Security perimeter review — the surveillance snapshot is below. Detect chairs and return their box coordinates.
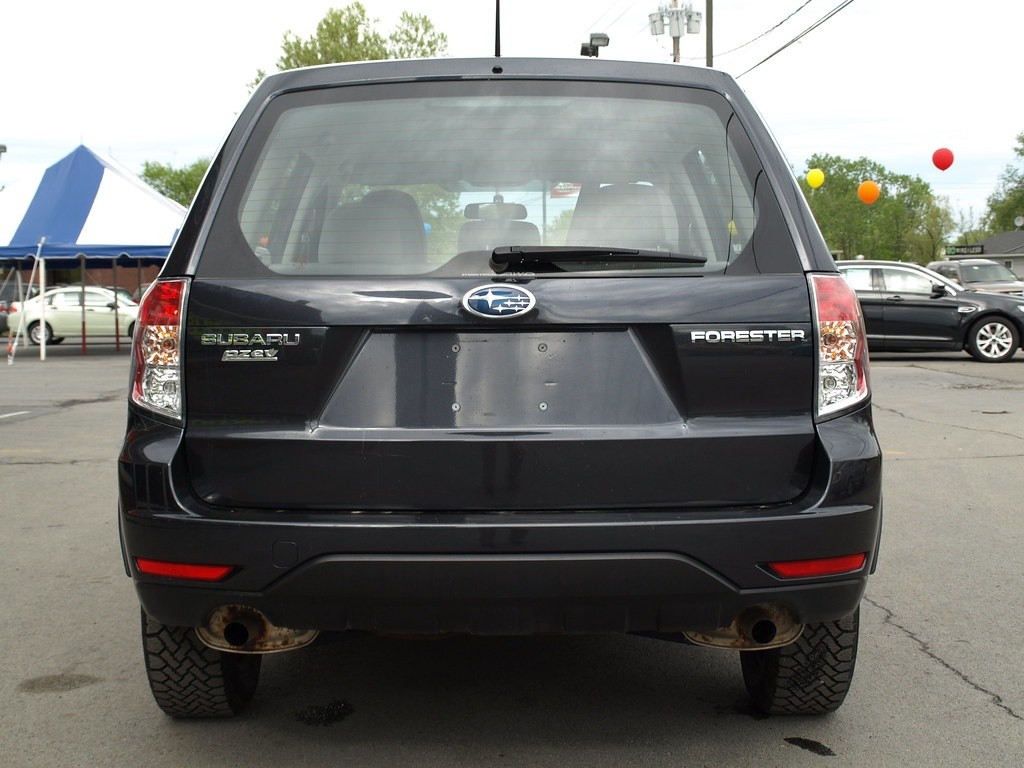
[317,190,427,270]
[570,182,682,254]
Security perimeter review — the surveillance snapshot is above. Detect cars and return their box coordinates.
[105,285,135,303]
[133,281,154,303]
[6,286,140,345]
[834,259,1024,364]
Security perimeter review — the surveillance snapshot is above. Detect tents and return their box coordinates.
[0,145,189,363]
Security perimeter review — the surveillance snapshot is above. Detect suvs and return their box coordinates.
[118,55,884,722]
[926,257,1024,300]
[0,281,40,337]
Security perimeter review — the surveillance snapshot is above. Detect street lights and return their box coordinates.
[648,0,702,65]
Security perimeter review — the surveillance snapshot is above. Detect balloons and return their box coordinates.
[858,181,881,205]
[806,169,825,189]
[933,148,954,171]
